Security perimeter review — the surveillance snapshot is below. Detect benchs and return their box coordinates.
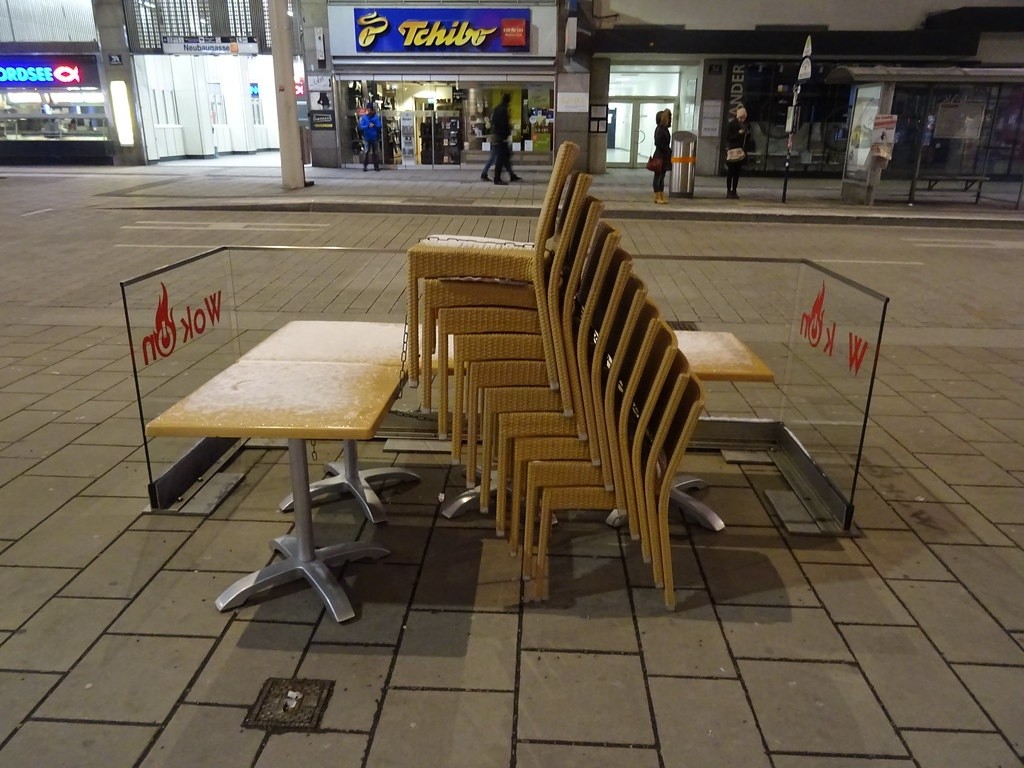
[923,174,990,192]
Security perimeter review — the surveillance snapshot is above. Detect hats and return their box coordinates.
[736,104,747,118]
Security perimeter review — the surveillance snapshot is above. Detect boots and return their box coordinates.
[655,191,668,204]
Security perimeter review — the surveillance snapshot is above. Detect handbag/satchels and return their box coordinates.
[726,148,745,161]
[646,156,663,172]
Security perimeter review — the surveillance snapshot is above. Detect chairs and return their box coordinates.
[405,141,711,616]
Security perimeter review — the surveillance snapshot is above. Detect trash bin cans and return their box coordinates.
[669,131,697,198]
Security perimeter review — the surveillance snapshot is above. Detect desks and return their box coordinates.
[603,327,776,531]
[144,319,431,624]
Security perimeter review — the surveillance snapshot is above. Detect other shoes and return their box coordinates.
[480,172,491,181]
[510,175,523,182]
[374,166,380,171]
[363,166,367,171]
[493,175,508,185]
[726,189,739,199]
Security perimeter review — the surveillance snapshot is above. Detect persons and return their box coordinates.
[652,108,672,205]
[359,102,382,172]
[724,104,752,200]
[480,92,524,185]
[69,119,76,132]
[42,118,58,138]
